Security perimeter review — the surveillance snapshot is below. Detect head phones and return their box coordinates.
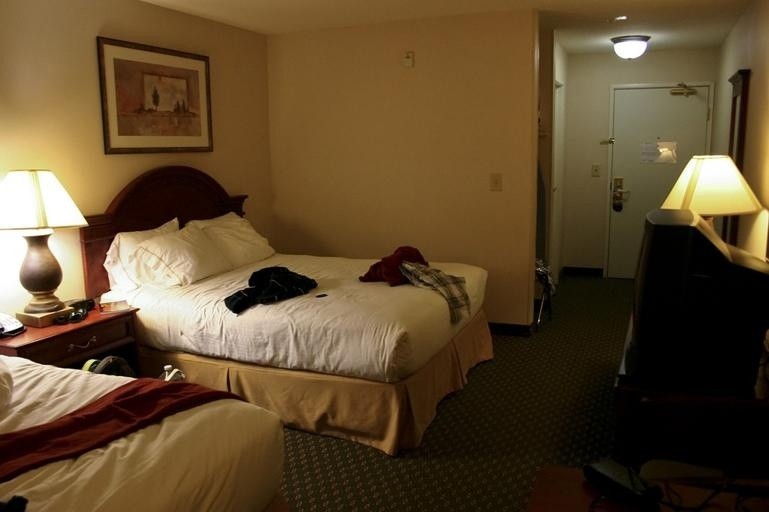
[52,307,88,325]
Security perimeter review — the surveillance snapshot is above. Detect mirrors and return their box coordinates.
[721,67,750,245]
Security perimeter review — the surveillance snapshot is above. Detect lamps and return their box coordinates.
[611,36,651,61]
[0,171,90,329]
[659,155,764,231]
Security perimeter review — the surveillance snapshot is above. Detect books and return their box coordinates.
[97,299,130,315]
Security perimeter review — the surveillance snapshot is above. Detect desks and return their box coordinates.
[526,463,768,512]
[612,312,768,473]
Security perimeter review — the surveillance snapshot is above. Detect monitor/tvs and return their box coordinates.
[625,209,769,396]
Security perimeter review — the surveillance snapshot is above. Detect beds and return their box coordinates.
[79,166,495,458]
[0,354,290,512]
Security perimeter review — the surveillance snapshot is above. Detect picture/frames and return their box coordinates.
[96,37,215,155]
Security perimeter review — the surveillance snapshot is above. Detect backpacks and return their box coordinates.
[81,357,139,379]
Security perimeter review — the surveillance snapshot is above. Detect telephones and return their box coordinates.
[0,312,24,338]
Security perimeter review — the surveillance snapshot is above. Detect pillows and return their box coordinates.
[103,210,277,291]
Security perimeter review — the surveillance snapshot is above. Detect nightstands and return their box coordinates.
[0,305,139,368]
